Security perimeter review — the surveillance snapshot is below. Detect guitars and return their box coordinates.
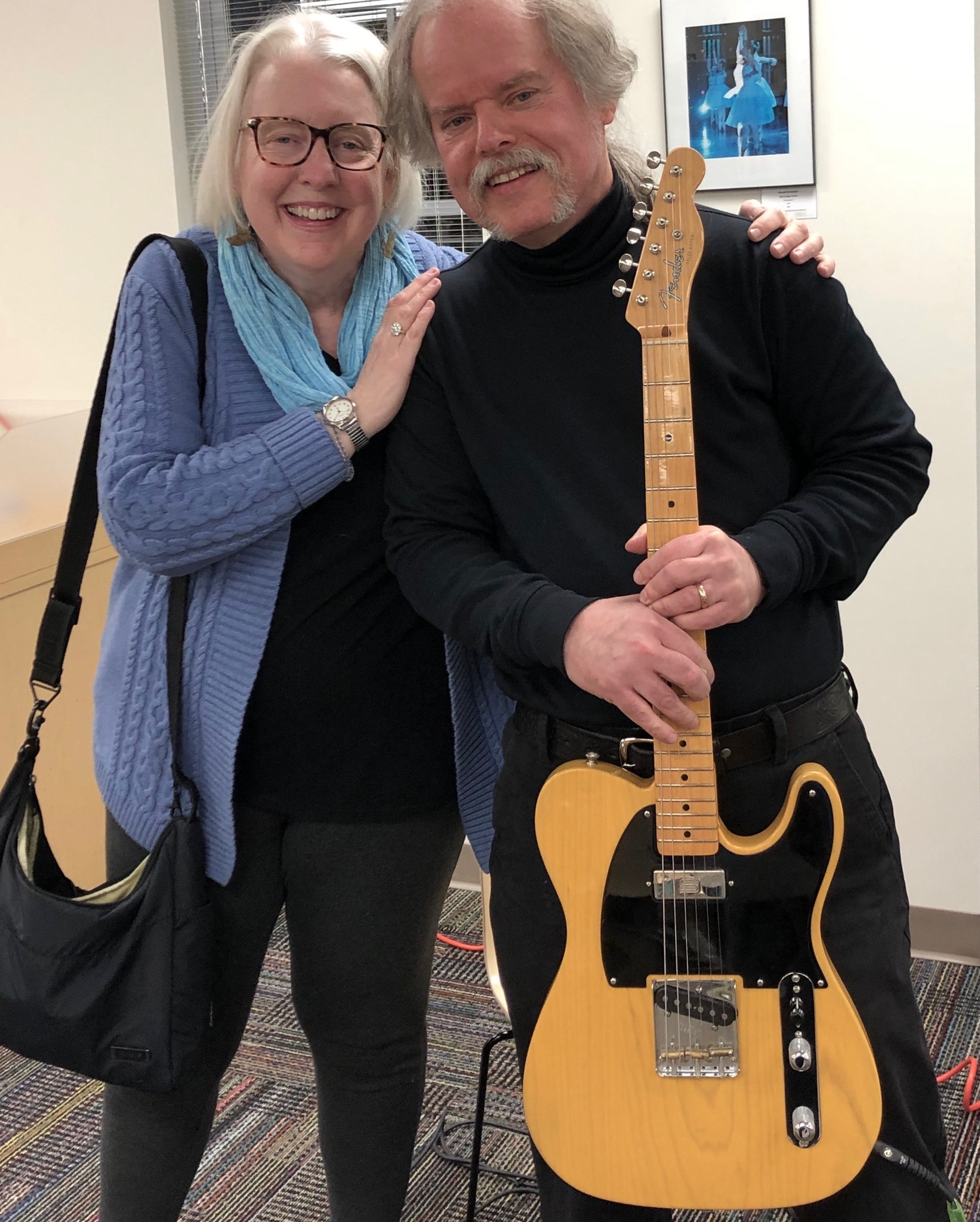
[517,144,881,1211]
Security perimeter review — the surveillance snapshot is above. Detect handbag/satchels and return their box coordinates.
[1,700,211,1092]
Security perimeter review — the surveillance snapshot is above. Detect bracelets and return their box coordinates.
[332,427,354,482]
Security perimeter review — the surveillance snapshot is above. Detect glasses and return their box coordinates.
[238,115,390,171]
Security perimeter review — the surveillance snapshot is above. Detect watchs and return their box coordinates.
[321,395,367,451]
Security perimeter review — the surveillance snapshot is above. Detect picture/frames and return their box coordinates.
[660,0,815,192]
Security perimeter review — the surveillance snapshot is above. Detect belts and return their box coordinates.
[516,671,857,779]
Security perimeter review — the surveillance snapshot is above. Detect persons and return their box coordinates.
[93,11,836,1222]
[368,0,947,1222]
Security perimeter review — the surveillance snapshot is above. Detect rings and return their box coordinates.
[390,322,406,335]
[696,583,708,608]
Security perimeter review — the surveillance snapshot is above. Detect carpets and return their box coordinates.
[1,882,980,1221]
